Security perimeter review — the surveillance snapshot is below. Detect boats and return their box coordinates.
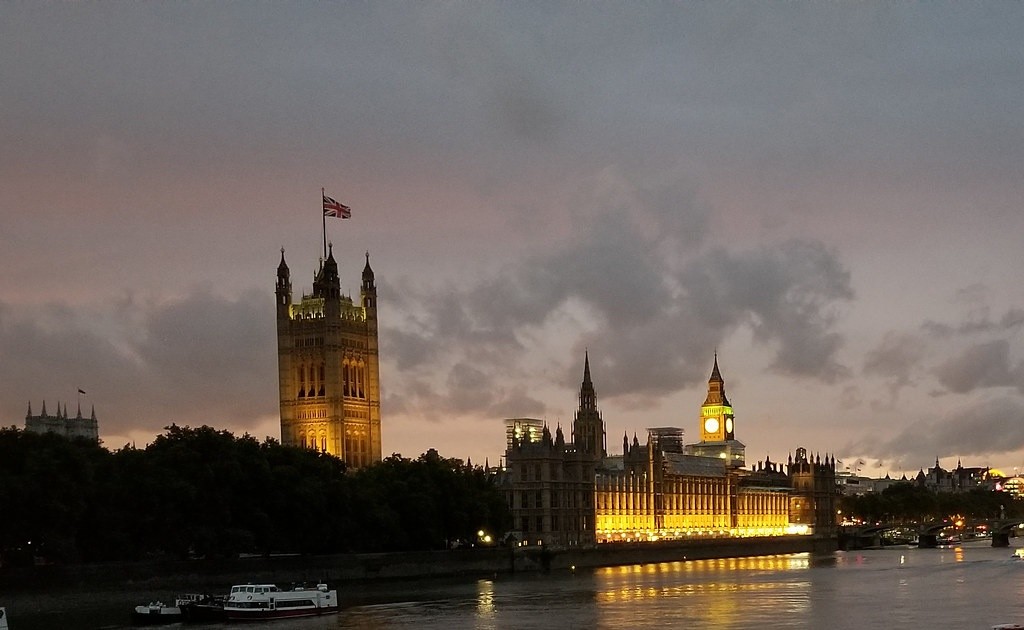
[178,596,227,625]
[134,594,203,624]
[223,583,338,622]
[908,540,919,545]
[939,535,961,545]
[1010,549,1024,560]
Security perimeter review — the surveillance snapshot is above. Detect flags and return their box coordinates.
[324,196,351,219]
[78,389,85,394]
